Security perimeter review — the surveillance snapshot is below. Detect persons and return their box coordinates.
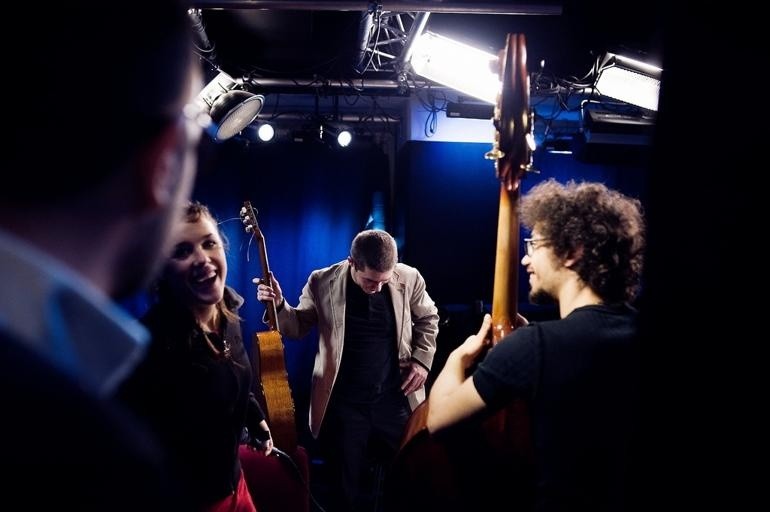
[426,176,683,510]
[0,0,209,510]
[141,195,274,512]
[252,228,440,511]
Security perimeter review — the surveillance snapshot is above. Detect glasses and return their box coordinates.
[523,237,551,256]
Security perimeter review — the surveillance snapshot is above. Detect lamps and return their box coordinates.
[592,52,664,112]
[187,50,356,154]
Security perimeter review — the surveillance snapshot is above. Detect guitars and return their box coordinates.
[484,32,531,348]
[240,200,295,440]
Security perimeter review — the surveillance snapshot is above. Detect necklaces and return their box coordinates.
[202,329,235,366]
